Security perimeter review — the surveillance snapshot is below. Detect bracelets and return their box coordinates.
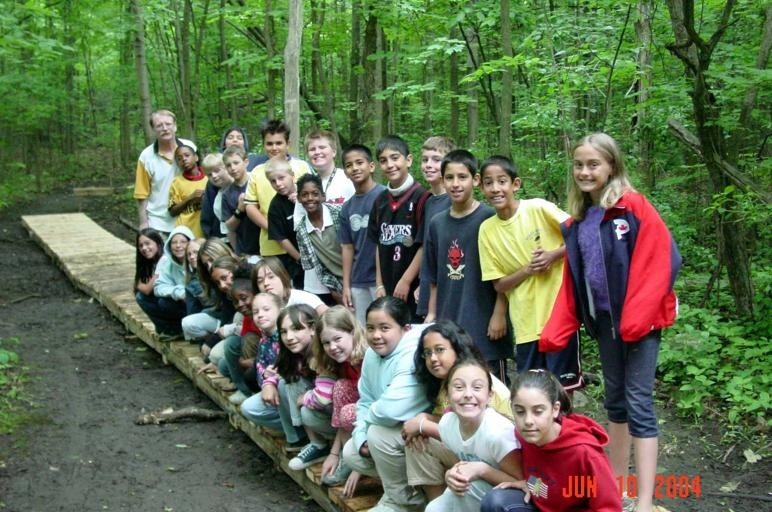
[419,418,429,435]
[375,284,384,291]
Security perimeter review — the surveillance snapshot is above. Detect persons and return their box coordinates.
[339,144,389,327]
[420,149,514,388]
[480,368,623,512]
[477,154,585,413]
[402,318,516,502]
[367,134,428,323]
[132,109,355,472]
[413,135,454,316]
[424,357,524,512]
[559,131,684,512]
[308,304,372,498]
[342,294,434,512]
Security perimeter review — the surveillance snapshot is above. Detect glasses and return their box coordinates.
[420,346,449,359]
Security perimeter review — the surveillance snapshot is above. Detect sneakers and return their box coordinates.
[353,478,384,497]
[158,331,184,342]
[622,497,660,512]
[368,504,394,512]
[222,379,256,405]
[286,437,309,452]
[322,453,353,487]
[289,442,331,471]
